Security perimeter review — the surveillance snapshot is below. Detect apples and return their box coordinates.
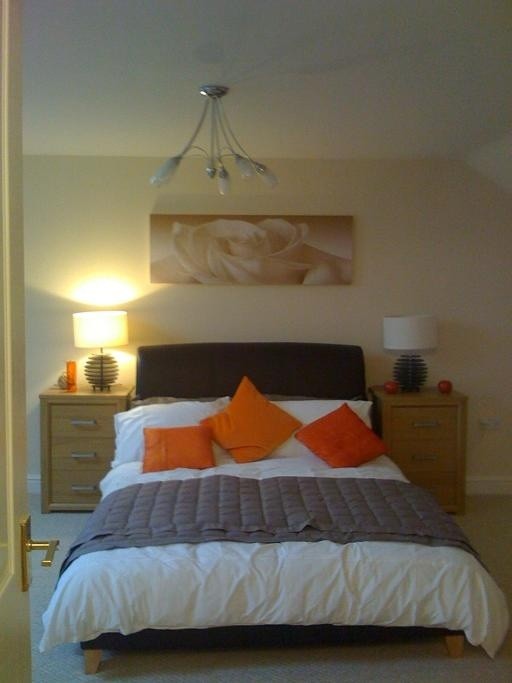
[438,380,452,394]
[383,381,398,394]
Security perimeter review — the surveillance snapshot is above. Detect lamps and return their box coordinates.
[149,85,276,197]
[73,310,130,390]
[382,312,437,392]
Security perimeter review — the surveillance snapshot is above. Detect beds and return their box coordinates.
[40,342,511,675]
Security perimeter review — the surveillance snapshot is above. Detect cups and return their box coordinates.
[66,360,77,393]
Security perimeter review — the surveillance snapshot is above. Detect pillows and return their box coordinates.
[110,397,229,468]
[270,400,373,457]
[295,403,392,467]
[143,427,216,472]
[200,376,302,463]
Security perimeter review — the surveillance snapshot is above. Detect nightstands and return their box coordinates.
[39,384,131,514]
[369,386,467,515]
